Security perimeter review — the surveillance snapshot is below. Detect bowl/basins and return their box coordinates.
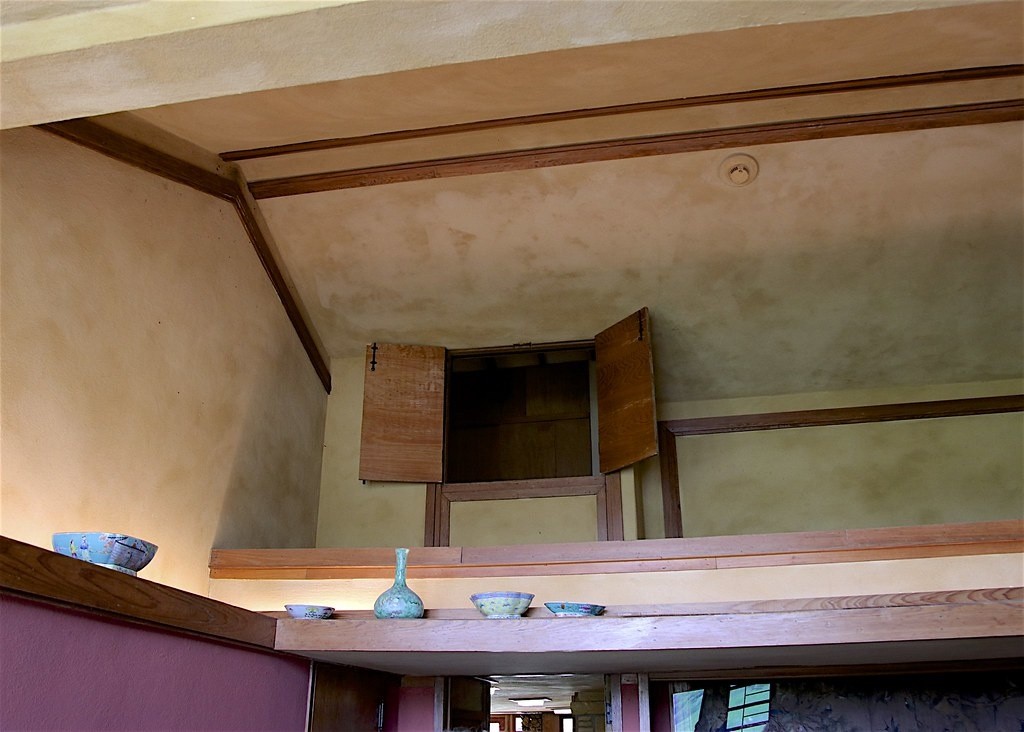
[51,532,159,578]
[284,604,335,620]
[468,591,535,619]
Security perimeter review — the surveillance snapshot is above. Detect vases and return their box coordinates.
[373,548,424,619]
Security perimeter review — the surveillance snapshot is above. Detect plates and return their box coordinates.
[544,602,606,617]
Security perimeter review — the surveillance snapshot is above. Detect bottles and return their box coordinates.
[374,547,424,619]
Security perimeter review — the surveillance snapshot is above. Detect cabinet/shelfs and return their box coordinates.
[358,306,658,547]
